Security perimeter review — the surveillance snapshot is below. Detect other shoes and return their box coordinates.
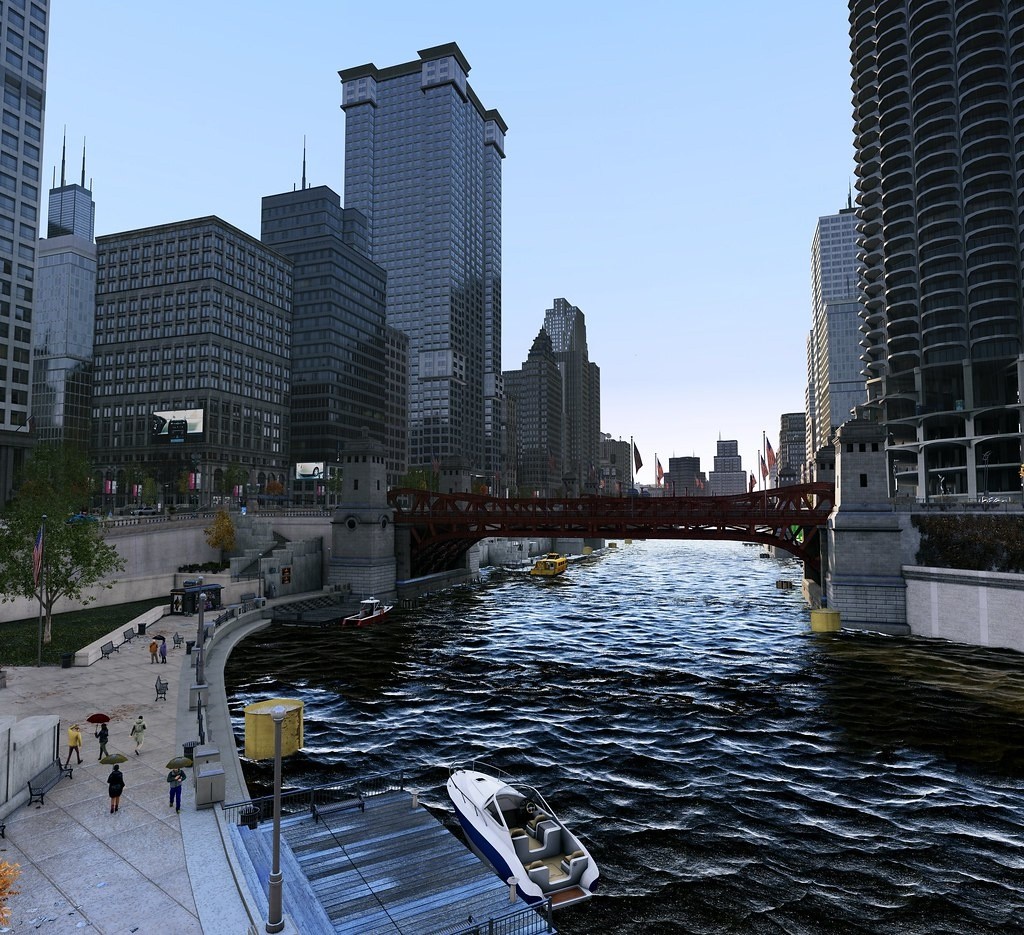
[78,760,83,764]
[170,803,173,807]
[98,757,101,760]
[176,810,180,814]
[135,750,140,755]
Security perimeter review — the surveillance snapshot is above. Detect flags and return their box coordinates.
[761,456,768,481]
[634,443,642,475]
[695,475,704,489]
[657,461,663,487]
[766,438,776,472]
[33,527,42,588]
[752,474,757,487]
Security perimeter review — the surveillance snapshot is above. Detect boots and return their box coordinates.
[115,805,118,811]
[111,805,114,813]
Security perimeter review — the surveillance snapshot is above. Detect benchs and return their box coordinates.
[27,757,74,806]
[155,676,169,701]
[173,632,185,650]
[101,641,120,660]
[311,793,365,824]
[241,592,256,604]
[123,628,139,644]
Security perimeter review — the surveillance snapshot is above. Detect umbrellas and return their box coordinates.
[86,714,110,723]
[166,756,192,770]
[100,754,129,766]
[153,635,165,640]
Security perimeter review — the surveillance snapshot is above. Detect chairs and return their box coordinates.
[0,819,6,839]
[524,860,544,872]
[528,814,547,831]
[510,828,526,838]
[564,850,584,865]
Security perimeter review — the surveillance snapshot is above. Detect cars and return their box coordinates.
[66,514,99,524]
[130,506,158,516]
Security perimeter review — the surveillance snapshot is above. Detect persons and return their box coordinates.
[160,640,167,664]
[64,724,83,769]
[150,640,158,664]
[107,765,125,813]
[131,716,147,755]
[167,768,187,814]
[94,724,109,760]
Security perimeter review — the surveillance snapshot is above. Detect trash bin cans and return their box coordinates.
[61,653,72,668]
[182,741,200,768]
[186,641,196,654]
[138,623,146,635]
[240,806,260,830]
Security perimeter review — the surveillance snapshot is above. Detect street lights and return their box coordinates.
[164,483,170,507]
[40,514,49,667]
[190,453,201,518]
[195,576,204,649]
[258,552,263,598]
[198,593,207,685]
[132,471,142,505]
[264,707,289,933]
[107,465,117,513]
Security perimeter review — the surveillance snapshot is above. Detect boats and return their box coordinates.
[529,552,569,578]
[343,588,394,627]
[444,756,602,912]
